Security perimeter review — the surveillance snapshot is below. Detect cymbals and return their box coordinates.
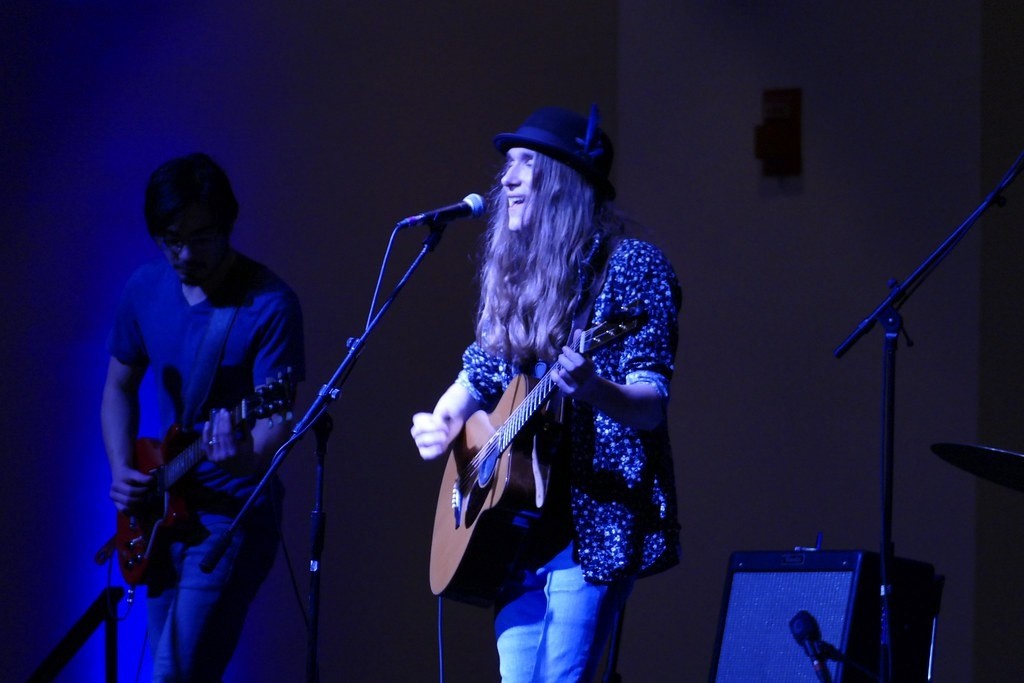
[927,438,1024,492]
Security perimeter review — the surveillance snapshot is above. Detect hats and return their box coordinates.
[493,101,620,206]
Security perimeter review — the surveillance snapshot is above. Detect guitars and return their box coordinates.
[429,299,638,596]
[118,369,299,585]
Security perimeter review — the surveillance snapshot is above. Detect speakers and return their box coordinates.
[708,545,945,683]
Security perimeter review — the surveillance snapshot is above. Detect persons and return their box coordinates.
[409,101,683,683]
[97,153,308,683]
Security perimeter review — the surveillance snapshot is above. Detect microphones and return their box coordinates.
[789,610,832,683]
[397,194,487,229]
